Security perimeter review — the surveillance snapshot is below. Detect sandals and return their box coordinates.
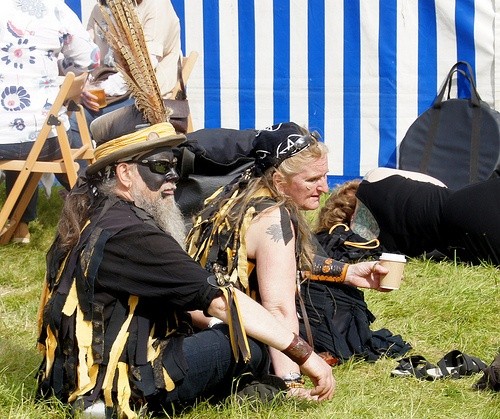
[391,355,435,378]
[427,350,487,379]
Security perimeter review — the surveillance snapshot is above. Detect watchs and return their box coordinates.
[281,372,301,380]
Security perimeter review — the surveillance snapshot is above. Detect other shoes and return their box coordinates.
[228,383,282,410]
[11,237,30,244]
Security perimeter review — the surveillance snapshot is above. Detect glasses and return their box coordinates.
[114,158,178,175]
[275,130,321,168]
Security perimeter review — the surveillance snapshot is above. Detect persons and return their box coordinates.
[33,147,336,419]
[0,0,101,243]
[185,122,404,402]
[55,0,181,192]
[318,167,500,269]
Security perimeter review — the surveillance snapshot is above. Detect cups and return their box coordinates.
[379,253,406,290]
[87,80,107,108]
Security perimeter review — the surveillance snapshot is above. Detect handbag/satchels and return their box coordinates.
[399,61,500,187]
[173,128,259,179]
[164,99,189,133]
[317,224,382,262]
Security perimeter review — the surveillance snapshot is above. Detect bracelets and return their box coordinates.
[284,378,305,388]
[281,331,314,367]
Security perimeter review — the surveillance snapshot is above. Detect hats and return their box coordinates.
[252,122,316,173]
[85,0,187,175]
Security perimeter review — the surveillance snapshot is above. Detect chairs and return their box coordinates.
[167,50,200,134]
[0,69,99,246]
